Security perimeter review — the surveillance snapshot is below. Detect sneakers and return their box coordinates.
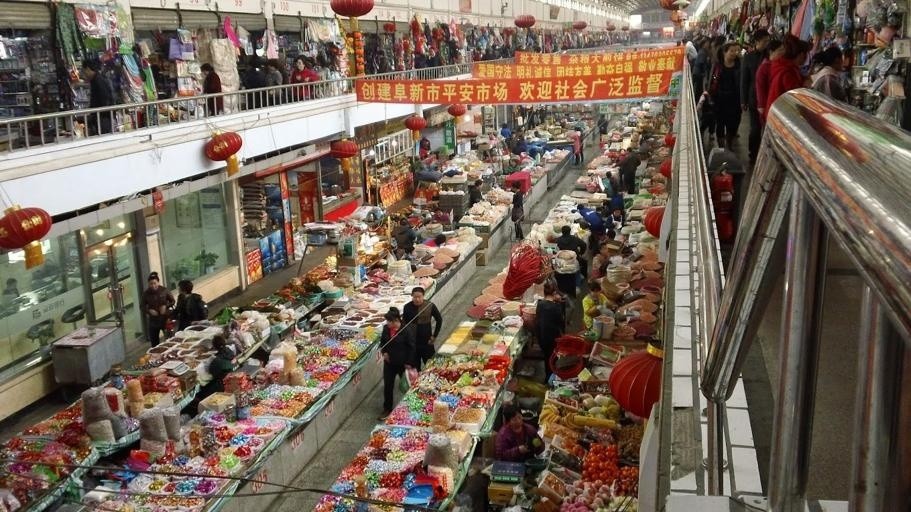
[516,239,524,242]
[378,409,391,421]
[512,238,519,241]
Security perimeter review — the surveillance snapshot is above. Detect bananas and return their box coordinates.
[540,402,584,431]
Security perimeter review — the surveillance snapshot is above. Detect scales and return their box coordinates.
[327,301,350,312]
[235,364,263,380]
[490,461,526,483]
[158,361,193,376]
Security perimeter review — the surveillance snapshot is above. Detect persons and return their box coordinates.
[757,40,784,113]
[808,44,849,103]
[604,172,618,192]
[544,31,637,50]
[422,235,446,248]
[576,203,602,250]
[83,57,116,137]
[612,147,641,194]
[198,63,224,114]
[3,278,19,297]
[569,127,585,165]
[598,114,609,141]
[509,180,524,240]
[431,209,449,228]
[616,149,628,188]
[767,38,812,120]
[582,282,610,330]
[507,131,518,149]
[742,29,770,162]
[139,272,175,349]
[470,179,484,207]
[547,225,588,281]
[599,231,616,248]
[401,286,442,369]
[495,404,545,463]
[376,29,539,71]
[502,123,511,138]
[209,337,239,378]
[682,34,726,97]
[708,42,742,151]
[379,307,416,421]
[604,208,625,231]
[245,58,341,105]
[170,280,208,332]
[395,219,417,256]
[536,282,566,382]
[639,134,653,154]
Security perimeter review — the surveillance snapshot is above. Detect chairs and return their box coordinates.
[61,303,86,330]
[26,319,55,346]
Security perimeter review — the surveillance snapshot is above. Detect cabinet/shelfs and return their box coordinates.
[52,326,126,385]
[1,253,133,368]
[243,227,287,275]
[244,247,264,286]
[322,188,361,223]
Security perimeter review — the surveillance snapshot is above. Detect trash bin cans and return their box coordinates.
[707,147,745,219]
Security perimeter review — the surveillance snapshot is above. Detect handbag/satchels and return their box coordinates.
[512,203,524,221]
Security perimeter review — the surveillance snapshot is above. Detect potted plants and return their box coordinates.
[195,252,219,274]
[39,331,51,359]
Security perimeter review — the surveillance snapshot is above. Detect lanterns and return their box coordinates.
[516,14,535,30]
[659,156,671,175]
[622,26,628,32]
[329,0,375,30]
[669,98,678,106]
[447,103,468,122]
[669,11,688,26]
[660,0,690,18]
[572,20,587,30]
[404,113,427,138]
[644,206,665,235]
[607,338,665,421]
[333,138,358,173]
[204,130,244,178]
[668,115,676,125]
[664,133,677,149]
[606,24,615,34]
[1,206,52,268]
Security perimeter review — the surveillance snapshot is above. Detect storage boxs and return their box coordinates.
[321,307,350,320]
[198,392,236,413]
[538,471,565,502]
[442,180,468,192]
[167,370,198,393]
[337,256,359,267]
[476,250,488,266]
[480,234,491,249]
[487,482,518,505]
[591,341,620,367]
[439,201,468,215]
[340,265,360,285]
[439,193,469,205]
